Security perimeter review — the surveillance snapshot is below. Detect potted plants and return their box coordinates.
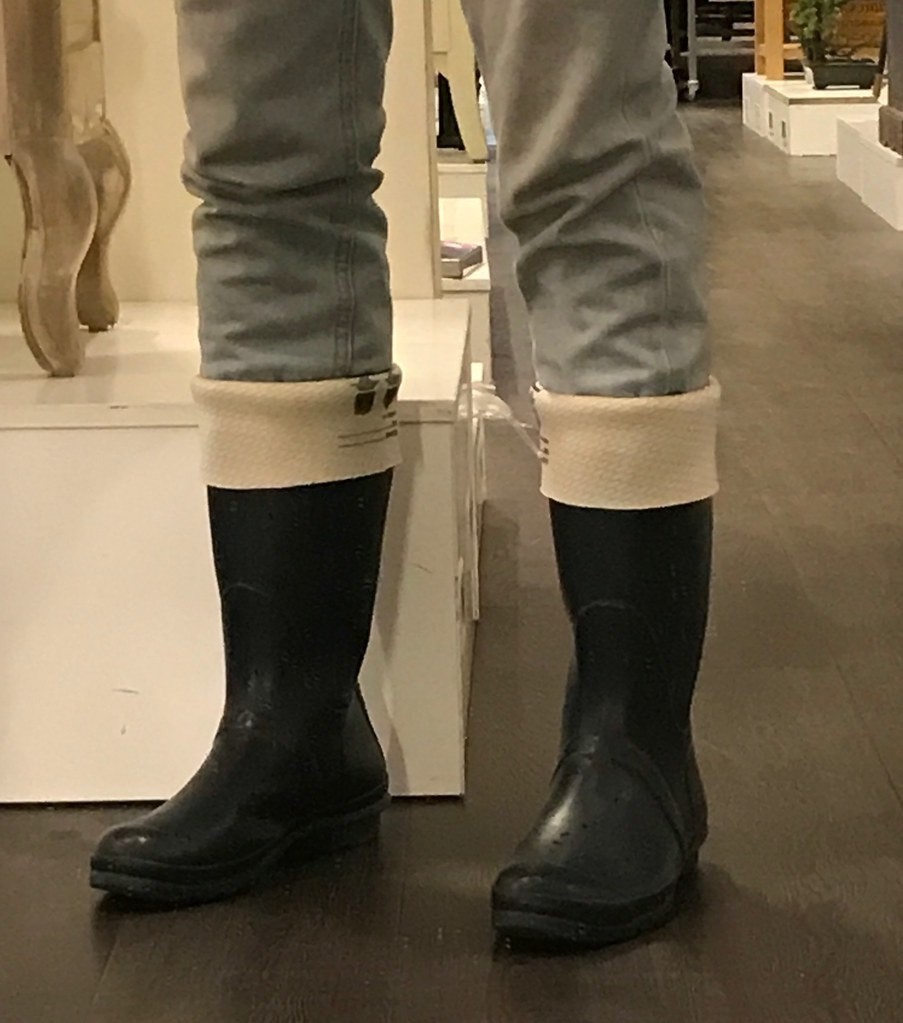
[788,0,880,90]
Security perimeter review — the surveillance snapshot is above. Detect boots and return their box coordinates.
[492,372,723,947]
[90,361,403,899]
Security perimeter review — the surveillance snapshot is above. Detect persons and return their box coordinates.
[87,0,720,946]
